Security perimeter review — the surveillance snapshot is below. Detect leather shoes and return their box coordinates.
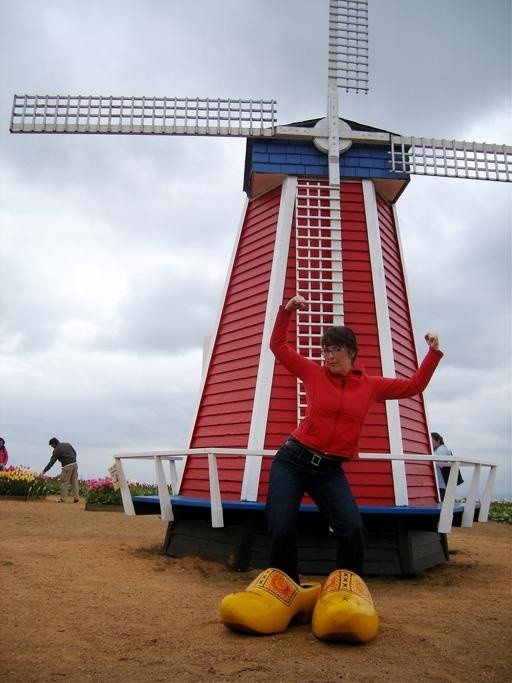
[220,569,321,636]
[312,570,378,644]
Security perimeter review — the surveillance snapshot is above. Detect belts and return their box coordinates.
[285,439,342,466]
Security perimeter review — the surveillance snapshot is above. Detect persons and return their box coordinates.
[0,437,8,471]
[264,295,444,586]
[40,437,80,503]
[431,433,464,502]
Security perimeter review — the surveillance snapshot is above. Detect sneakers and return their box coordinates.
[74,500,79,503]
[58,498,64,503]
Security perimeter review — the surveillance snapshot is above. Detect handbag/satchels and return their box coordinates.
[439,466,464,487]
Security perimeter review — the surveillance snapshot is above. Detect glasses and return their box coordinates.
[320,344,350,354]
